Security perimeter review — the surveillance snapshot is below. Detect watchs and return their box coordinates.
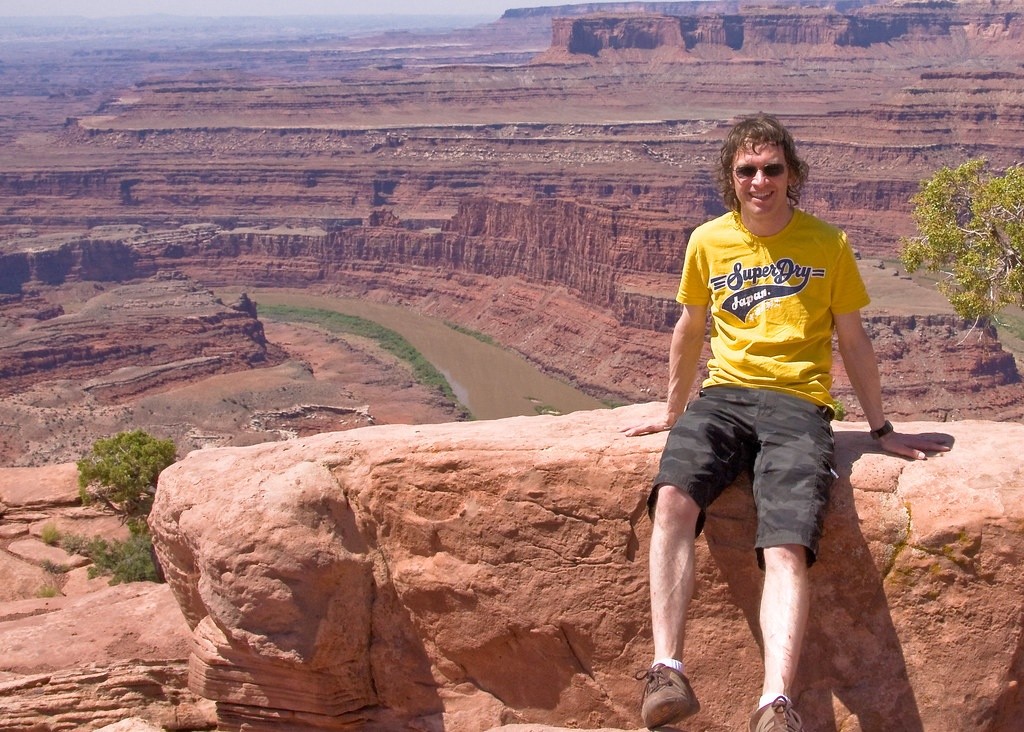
[870,420,893,440]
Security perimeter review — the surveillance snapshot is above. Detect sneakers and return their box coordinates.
[748,695,804,732]
[634,663,700,730]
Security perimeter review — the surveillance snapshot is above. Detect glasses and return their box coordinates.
[733,163,790,179]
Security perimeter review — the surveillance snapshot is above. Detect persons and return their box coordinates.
[620,120,954,732]
